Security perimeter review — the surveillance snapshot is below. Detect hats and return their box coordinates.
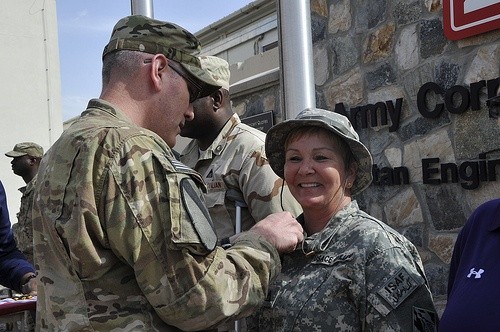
[4,142,44,159]
[101,15,222,101]
[197,54,231,92]
[266,107,373,199]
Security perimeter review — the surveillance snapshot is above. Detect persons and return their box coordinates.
[0,179,36,295]
[30,15,305,331]
[439,197,500,331]
[176,55,306,331]
[5,142,43,265]
[244,108,440,332]
